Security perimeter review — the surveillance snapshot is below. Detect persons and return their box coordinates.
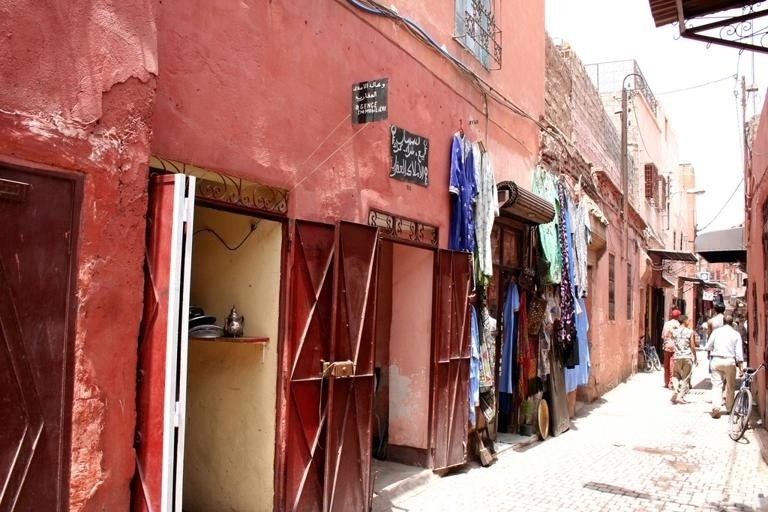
[697,302,749,418]
[661,308,683,387]
[668,315,700,403]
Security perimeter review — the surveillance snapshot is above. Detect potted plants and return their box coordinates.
[520,399,533,437]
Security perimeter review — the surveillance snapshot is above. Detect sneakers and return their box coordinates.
[671,393,689,404]
[711,409,721,419]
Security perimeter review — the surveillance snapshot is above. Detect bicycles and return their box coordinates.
[729,363,766,441]
[638,335,661,372]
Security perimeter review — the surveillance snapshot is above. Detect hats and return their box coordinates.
[672,309,681,318]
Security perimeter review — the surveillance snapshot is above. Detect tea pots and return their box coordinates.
[222,305,244,338]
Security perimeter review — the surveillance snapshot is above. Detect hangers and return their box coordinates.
[459,119,464,136]
[477,129,486,154]
